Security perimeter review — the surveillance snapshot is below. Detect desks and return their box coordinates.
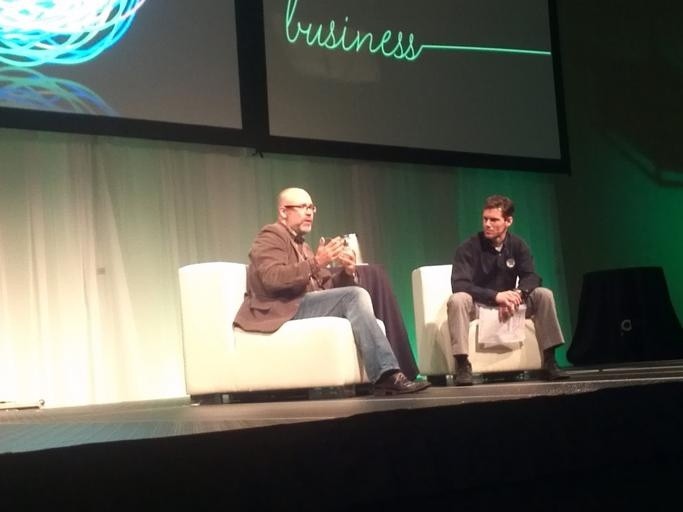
[324,264,420,381]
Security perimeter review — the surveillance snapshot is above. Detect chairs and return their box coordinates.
[176,261,387,402]
[410,263,541,377]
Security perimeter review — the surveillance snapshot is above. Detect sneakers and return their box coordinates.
[541,358,570,381]
[453,362,473,387]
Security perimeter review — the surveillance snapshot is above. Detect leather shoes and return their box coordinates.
[371,369,432,397]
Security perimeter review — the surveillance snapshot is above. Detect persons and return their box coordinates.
[231,187,432,397]
[445,195,570,387]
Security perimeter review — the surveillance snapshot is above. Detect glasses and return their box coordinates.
[284,203,316,213]
